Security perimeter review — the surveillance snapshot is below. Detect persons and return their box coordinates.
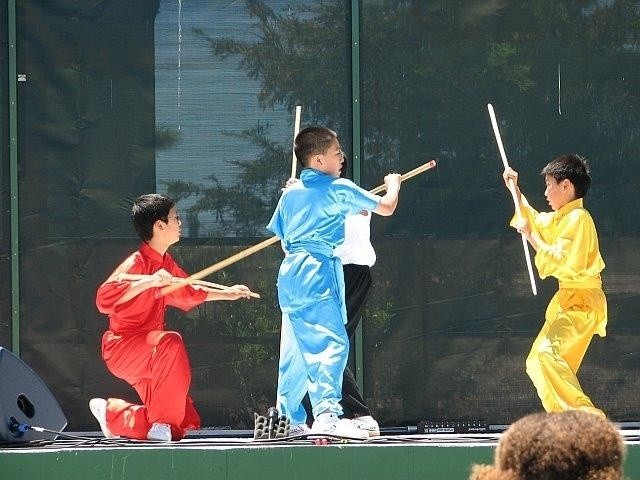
[266,125,404,441]
[279,147,382,440]
[501,151,608,421]
[88,192,252,443]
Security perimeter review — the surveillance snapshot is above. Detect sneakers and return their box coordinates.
[289,415,381,441]
[147,421,173,443]
[88,397,120,439]
